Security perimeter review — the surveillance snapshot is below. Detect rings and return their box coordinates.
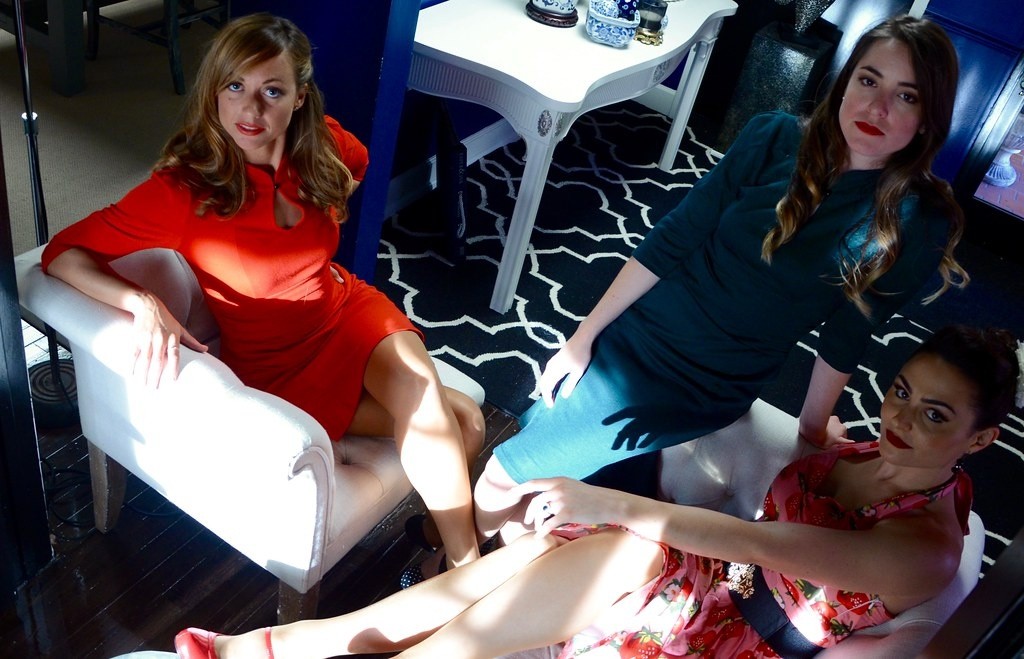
[543,504,552,515]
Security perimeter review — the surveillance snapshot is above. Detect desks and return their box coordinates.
[403,0,739,316]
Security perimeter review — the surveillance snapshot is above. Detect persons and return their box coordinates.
[41,14,480,570]
[174,326,1021,659]
[399,15,959,589]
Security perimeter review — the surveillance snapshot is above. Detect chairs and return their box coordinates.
[85,0,231,94]
[497,397,987,659]
[14,243,487,624]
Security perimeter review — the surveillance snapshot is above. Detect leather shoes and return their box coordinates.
[174,627,274,659]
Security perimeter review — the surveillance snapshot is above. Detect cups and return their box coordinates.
[637,0,669,36]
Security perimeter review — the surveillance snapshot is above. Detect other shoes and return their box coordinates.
[405,515,436,555]
[400,541,492,590]
